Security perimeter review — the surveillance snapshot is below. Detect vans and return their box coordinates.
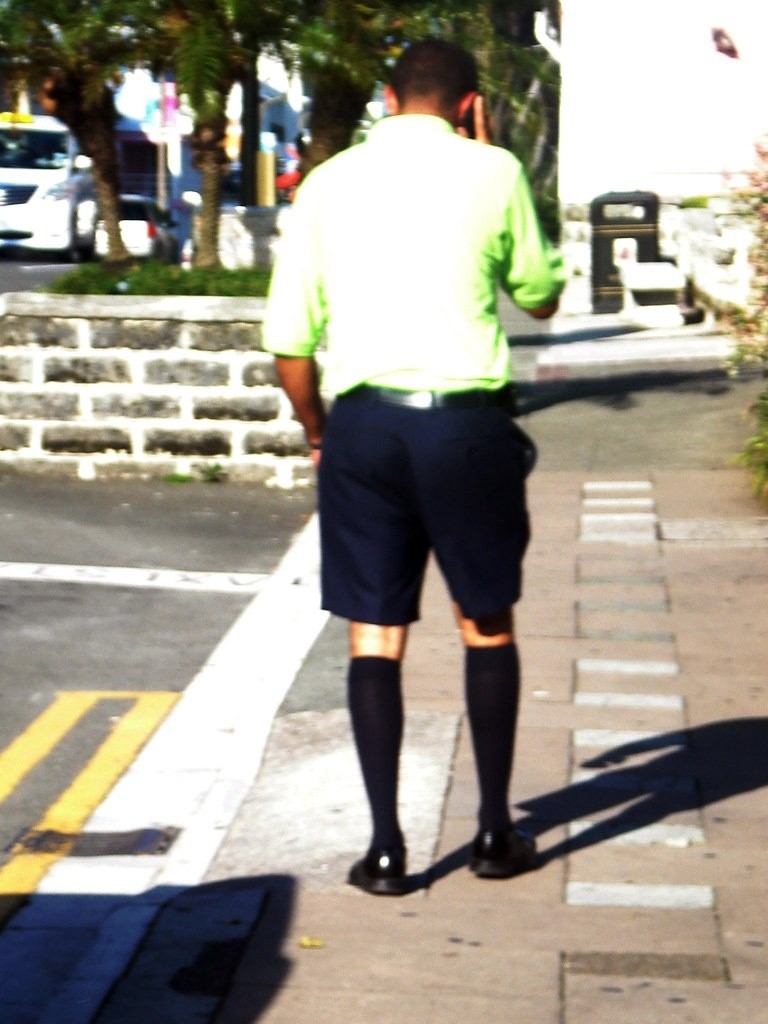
[0,112,95,261]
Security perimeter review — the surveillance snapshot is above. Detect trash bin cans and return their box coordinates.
[586,190,660,312]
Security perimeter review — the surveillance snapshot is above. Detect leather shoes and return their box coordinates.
[350,848,406,894]
[471,828,535,875]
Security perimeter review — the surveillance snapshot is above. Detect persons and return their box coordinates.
[260,34,567,894]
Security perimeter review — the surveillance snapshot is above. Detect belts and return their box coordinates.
[339,386,515,411]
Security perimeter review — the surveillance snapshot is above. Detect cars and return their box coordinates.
[95,194,179,261]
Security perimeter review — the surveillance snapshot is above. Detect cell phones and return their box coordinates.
[462,103,475,141]
